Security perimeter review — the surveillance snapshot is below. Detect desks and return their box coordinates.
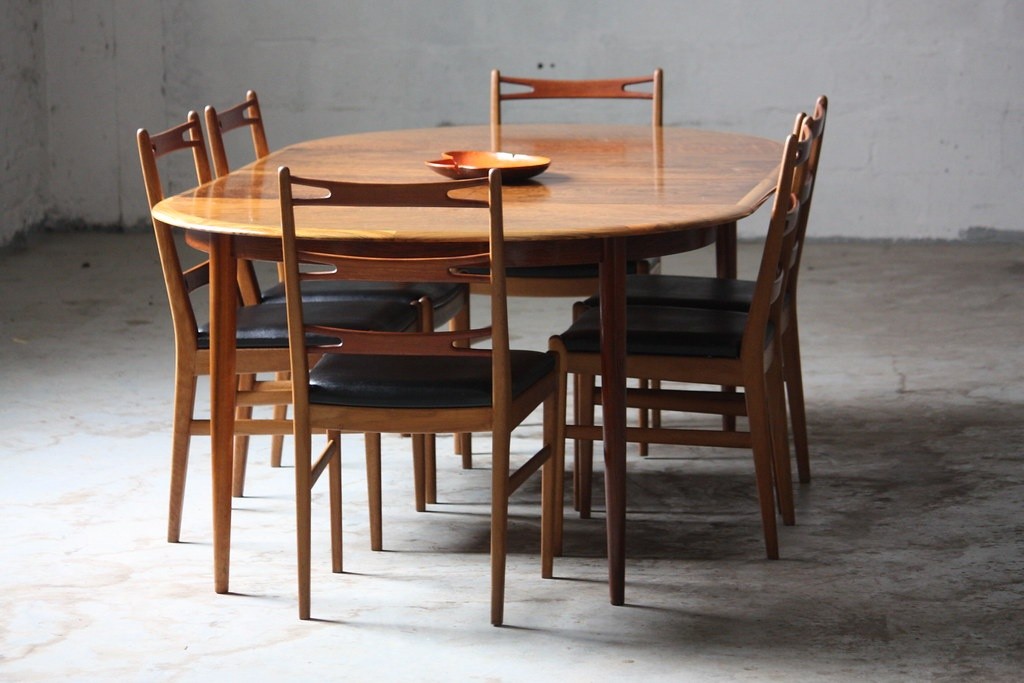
[152,123,785,606]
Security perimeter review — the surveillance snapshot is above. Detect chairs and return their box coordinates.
[137,68,830,627]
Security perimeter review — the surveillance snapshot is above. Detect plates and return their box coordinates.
[425,151,551,181]
